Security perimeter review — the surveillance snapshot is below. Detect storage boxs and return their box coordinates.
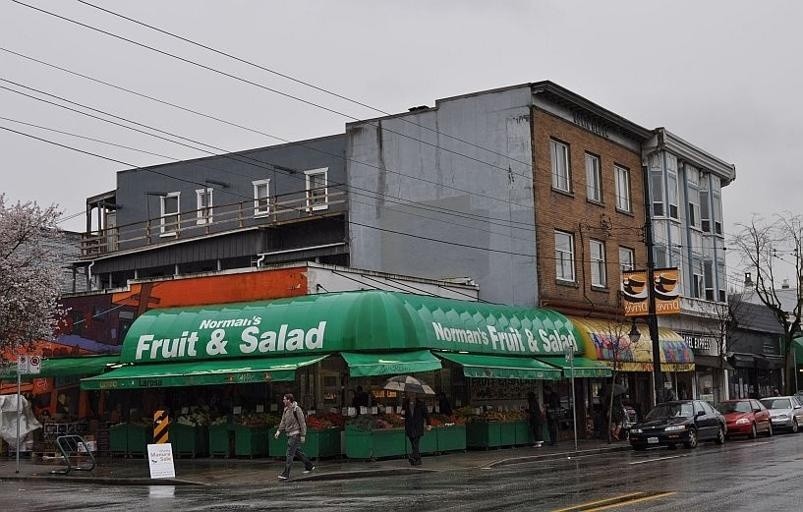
[77,440,98,452]
[18,440,33,451]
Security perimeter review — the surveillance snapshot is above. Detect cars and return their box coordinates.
[715,399,773,439]
[629,400,728,449]
[758,396,803,433]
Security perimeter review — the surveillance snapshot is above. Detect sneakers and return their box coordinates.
[278,475,289,480]
[302,466,315,474]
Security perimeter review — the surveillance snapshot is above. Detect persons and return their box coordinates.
[351,385,369,414]
[542,385,562,445]
[664,388,680,415]
[606,394,630,441]
[525,390,545,448]
[398,391,432,465]
[273,394,315,479]
[110,403,123,425]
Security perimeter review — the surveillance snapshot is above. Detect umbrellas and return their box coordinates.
[381,374,436,394]
[596,383,627,397]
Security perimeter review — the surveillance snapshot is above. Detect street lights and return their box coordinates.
[626,315,664,405]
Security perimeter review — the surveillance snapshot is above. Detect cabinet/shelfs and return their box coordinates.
[108,419,551,462]
